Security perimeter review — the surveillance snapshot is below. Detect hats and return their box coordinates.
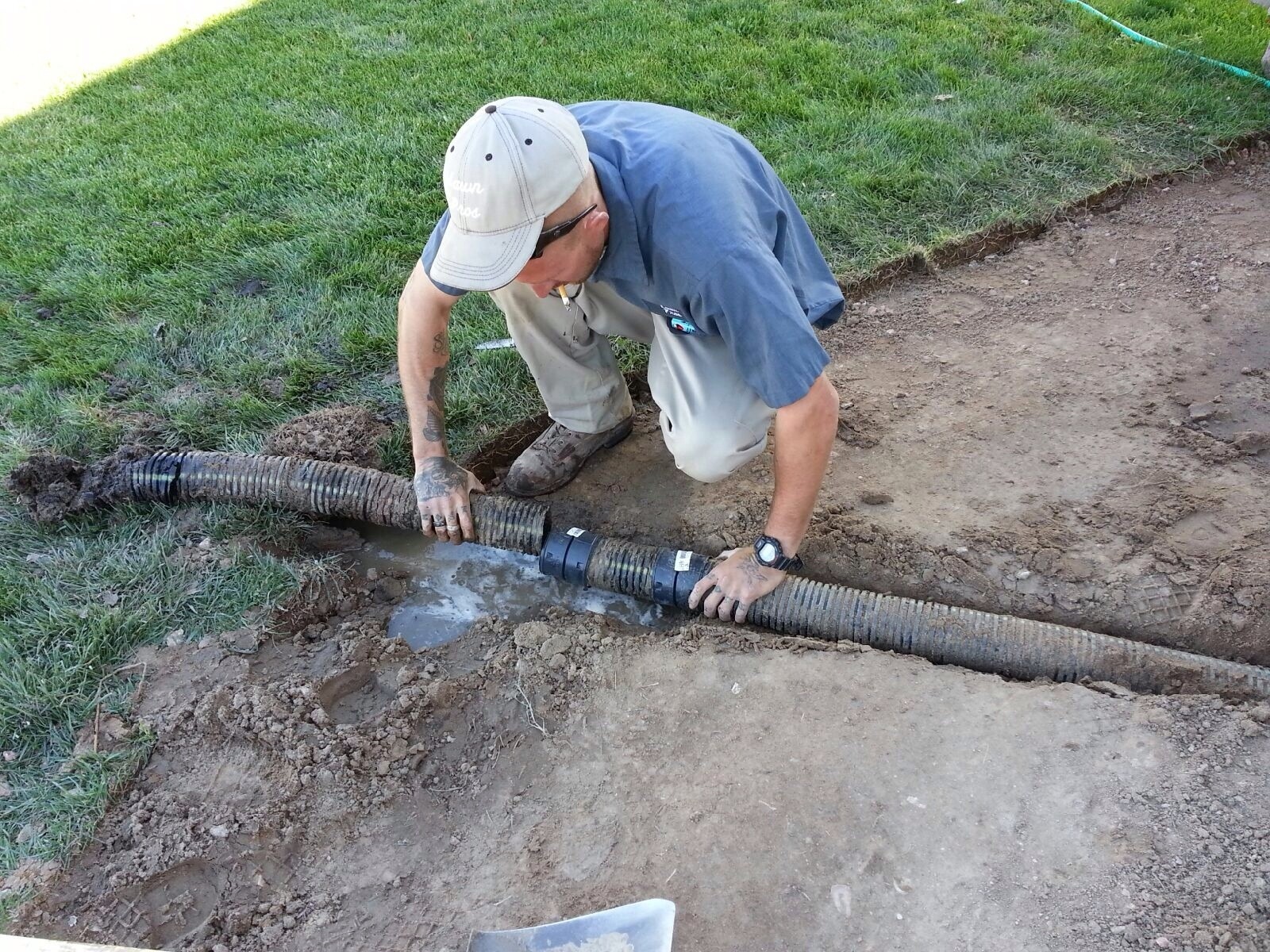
[429,96,589,292]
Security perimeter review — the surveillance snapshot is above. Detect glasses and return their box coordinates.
[525,202,597,259]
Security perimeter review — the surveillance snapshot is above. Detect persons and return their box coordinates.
[397,96,850,623]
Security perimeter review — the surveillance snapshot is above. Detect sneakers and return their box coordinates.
[505,407,636,496]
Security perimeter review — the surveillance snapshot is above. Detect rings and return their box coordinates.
[446,525,460,531]
[432,521,446,526]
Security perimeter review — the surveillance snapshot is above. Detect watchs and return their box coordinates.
[754,535,804,571]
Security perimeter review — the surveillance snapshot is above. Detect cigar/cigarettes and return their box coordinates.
[558,284,571,312]
